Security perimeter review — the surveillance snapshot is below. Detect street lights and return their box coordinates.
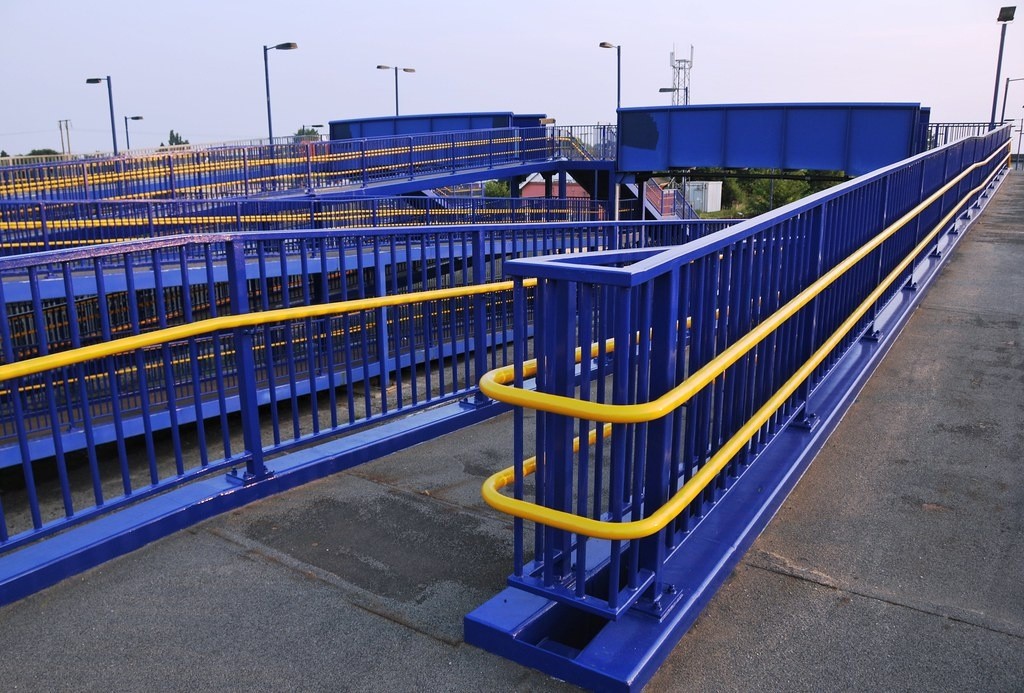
[302,125,325,137]
[86,75,118,154]
[989,6,1017,129]
[378,64,415,116]
[659,87,687,105]
[124,116,144,150]
[263,42,299,190]
[600,42,621,108]
